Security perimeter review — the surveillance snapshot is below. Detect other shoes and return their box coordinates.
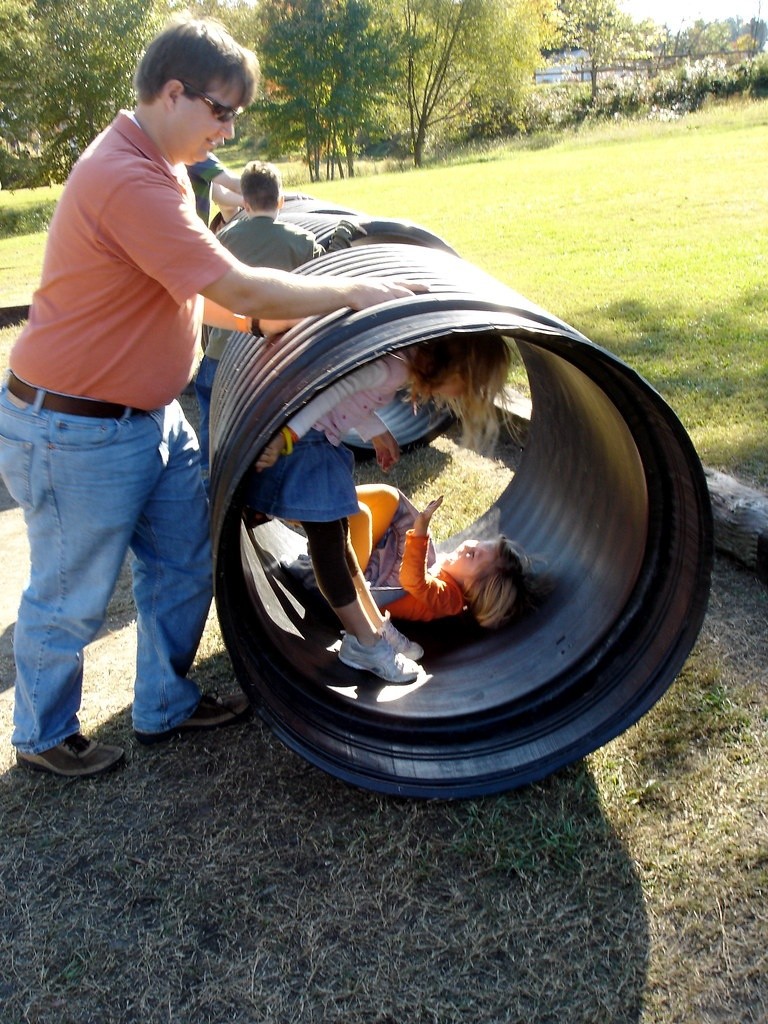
[280,548,313,570]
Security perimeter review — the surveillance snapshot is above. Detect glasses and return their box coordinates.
[182,78,244,122]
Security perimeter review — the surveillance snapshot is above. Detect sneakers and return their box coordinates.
[376,610,424,661]
[338,629,418,683]
[134,689,251,746]
[15,732,125,777]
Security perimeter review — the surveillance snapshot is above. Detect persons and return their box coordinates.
[183,154,529,685]
[1,20,426,778]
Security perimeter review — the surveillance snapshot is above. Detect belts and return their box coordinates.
[6,372,148,419]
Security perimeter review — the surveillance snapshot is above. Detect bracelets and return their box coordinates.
[233,315,248,334]
[251,319,264,338]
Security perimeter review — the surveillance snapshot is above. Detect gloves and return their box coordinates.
[328,216,360,250]
